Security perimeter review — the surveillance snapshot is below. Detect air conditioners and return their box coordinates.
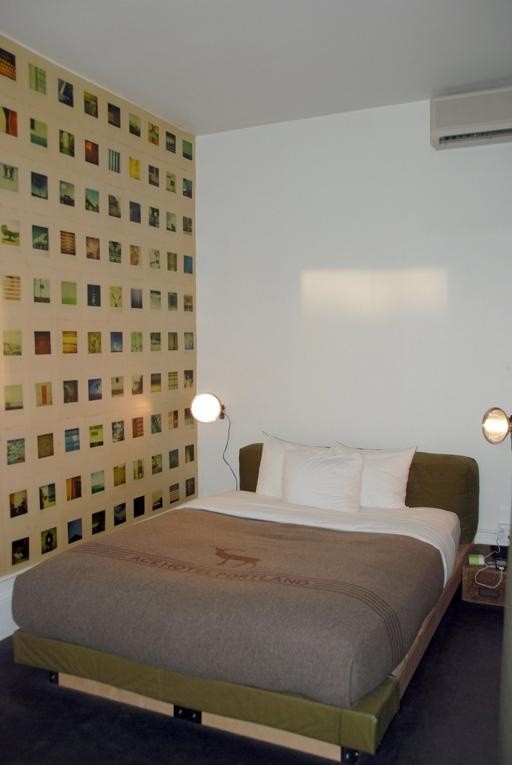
[430,86,511,151]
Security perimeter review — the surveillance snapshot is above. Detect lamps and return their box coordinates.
[190,394,225,424]
[482,407,512,445]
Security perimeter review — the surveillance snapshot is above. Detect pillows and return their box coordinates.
[256,431,332,499]
[334,441,417,508]
[282,451,362,512]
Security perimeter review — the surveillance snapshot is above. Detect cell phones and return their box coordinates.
[496,558,507,570]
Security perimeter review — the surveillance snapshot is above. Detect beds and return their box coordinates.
[14,444,479,765]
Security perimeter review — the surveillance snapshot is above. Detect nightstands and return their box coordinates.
[462,544,507,606]
